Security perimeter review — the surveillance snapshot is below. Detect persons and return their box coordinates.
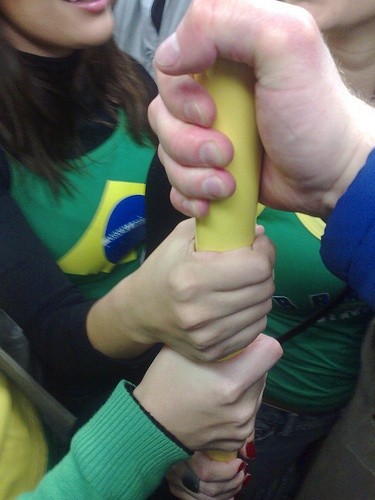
[0,0,273,417]
[147,0,375,309]
[101,0,196,84]
[0,332,285,500]
[188,0,375,500]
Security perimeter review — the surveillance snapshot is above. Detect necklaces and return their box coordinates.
[360,92,375,104]
